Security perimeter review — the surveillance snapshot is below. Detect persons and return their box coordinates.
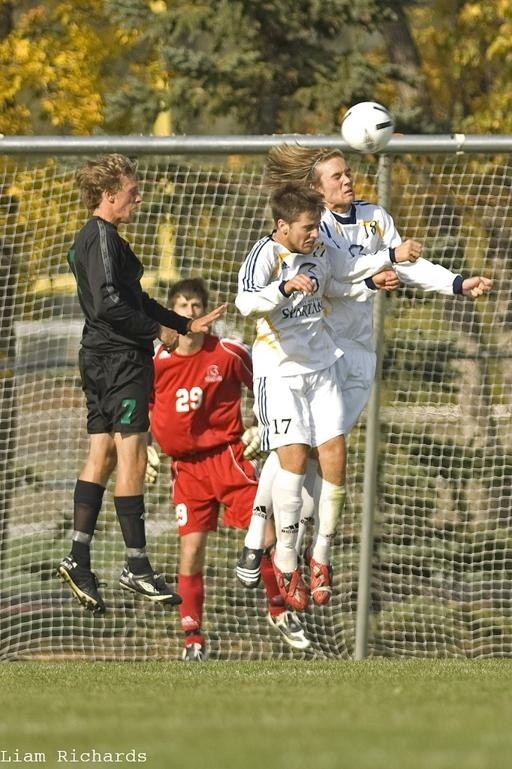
[238,140,498,591]
[55,153,228,616]
[146,278,314,663]
[233,186,425,613]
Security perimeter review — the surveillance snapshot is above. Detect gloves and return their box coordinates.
[143,445,161,484]
[241,425,262,460]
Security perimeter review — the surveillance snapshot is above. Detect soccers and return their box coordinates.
[341,101,393,153]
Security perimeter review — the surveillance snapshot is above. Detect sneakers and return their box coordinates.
[264,611,312,650]
[303,544,332,605]
[119,565,182,607]
[181,633,205,662]
[269,549,307,611]
[235,546,263,589]
[56,553,111,614]
